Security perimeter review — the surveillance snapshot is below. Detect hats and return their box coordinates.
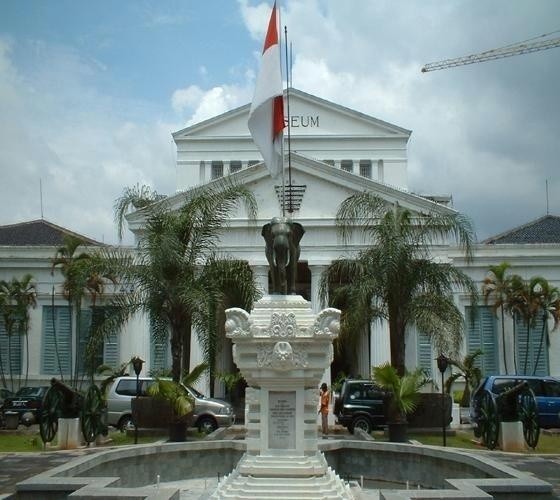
[320,383,327,389]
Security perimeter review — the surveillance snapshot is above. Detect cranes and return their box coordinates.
[421,28,560,75]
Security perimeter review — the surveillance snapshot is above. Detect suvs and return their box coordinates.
[333,379,453,435]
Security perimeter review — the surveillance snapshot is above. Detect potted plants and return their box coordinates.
[368,360,432,443]
[147,361,208,443]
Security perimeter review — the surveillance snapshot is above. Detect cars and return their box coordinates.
[0,386,50,424]
[468,375,560,429]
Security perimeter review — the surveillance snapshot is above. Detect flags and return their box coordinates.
[247,0,287,179]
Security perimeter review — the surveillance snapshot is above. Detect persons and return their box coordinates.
[318,382,330,435]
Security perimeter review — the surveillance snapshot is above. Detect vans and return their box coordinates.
[103,376,236,434]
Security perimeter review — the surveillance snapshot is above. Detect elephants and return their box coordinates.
[261,216,306,296]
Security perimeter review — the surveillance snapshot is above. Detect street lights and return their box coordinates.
[433,353,451,447]
[130,356,146,444]
